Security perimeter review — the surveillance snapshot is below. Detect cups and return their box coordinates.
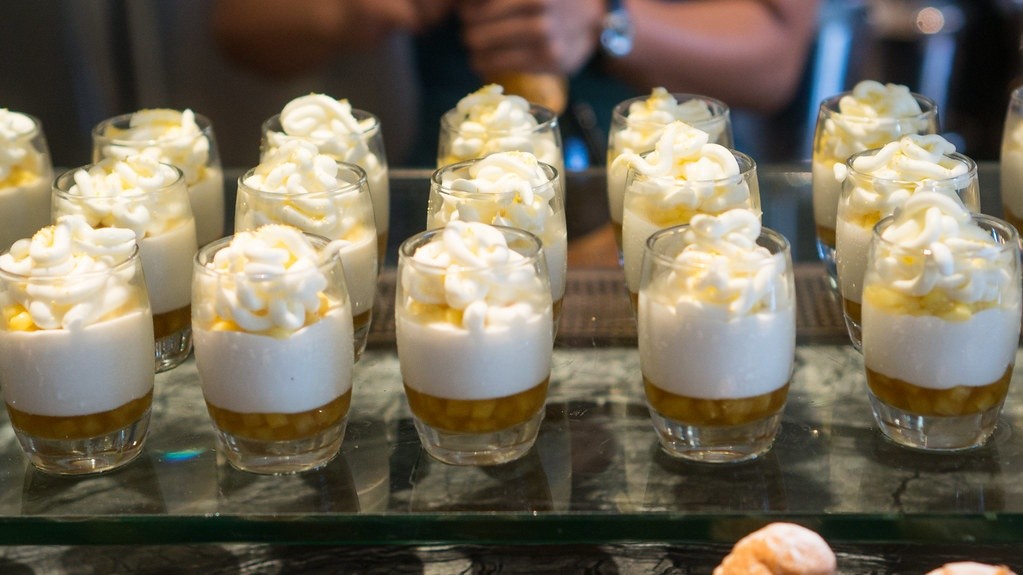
[0,79,1023,475]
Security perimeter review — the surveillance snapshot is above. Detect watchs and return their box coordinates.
[580,1,637,78]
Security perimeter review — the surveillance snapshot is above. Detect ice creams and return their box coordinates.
[0,79,1023,442]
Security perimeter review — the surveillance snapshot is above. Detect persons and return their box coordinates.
[214,2,819,164]
